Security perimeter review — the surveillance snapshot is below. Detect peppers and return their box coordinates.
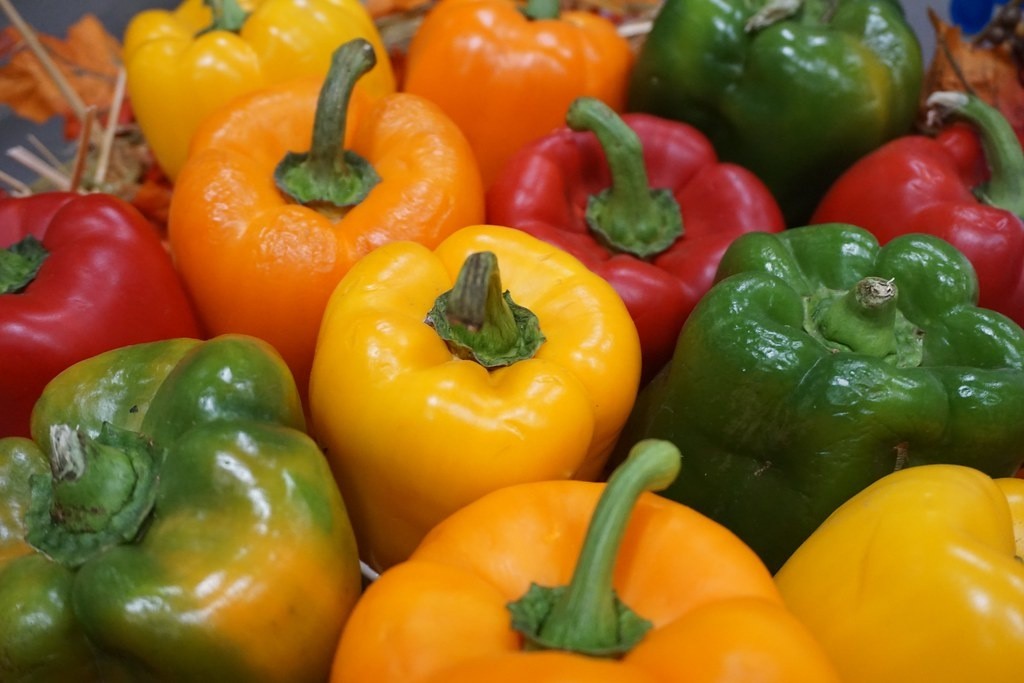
[0,0,1024,683]
